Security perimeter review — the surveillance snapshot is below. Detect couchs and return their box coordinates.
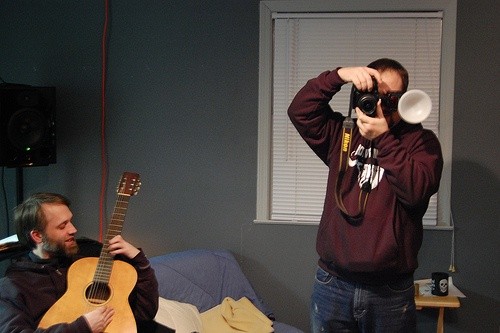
[152,248,306,333]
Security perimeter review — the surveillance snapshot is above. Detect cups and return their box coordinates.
[431,272,450,297]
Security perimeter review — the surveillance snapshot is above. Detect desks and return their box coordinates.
[414,296,461,333]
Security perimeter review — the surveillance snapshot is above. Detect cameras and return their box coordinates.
[352,78,431,123]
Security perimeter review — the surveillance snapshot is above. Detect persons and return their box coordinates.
[0,193,159,333]
[287,59,444,333]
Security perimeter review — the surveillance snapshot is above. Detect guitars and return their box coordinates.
[37,172,142,332]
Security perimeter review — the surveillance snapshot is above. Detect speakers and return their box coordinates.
[0,83,56,168]
